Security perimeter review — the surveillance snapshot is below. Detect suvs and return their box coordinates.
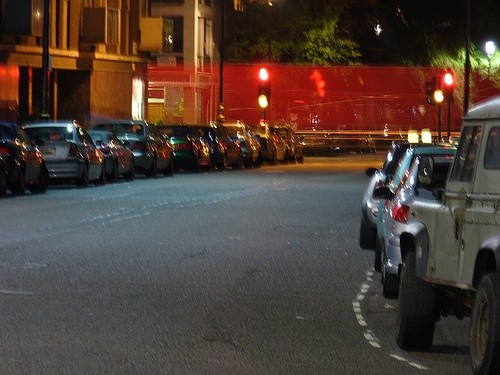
[397,99,500,375]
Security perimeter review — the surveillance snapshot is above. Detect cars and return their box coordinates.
[1,118,306,196]
[359,140,465,299]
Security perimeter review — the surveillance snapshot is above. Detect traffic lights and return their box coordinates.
[424,82,435,106]
[257,64,272,109]
[445,72,454,101]
[433,88,444,104]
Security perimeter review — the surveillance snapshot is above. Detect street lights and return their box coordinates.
[485,42,495,83]
